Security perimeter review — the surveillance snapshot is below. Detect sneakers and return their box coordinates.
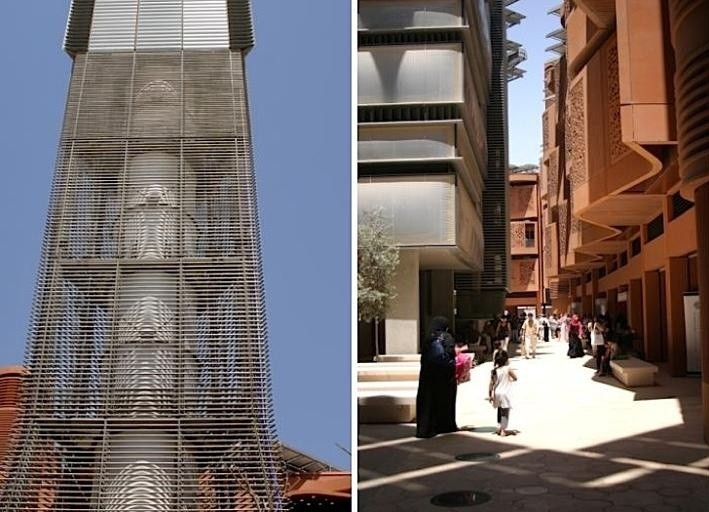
[496,429,512,437]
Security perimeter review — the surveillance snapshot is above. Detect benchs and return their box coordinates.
[608,351,657,388]
[357,354,422,424]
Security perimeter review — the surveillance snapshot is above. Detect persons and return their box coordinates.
[488,349,518,437]
[457,309,639,378]
[414,315,462,438]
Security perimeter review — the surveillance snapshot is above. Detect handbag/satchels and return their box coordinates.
[454,348,472,385]
